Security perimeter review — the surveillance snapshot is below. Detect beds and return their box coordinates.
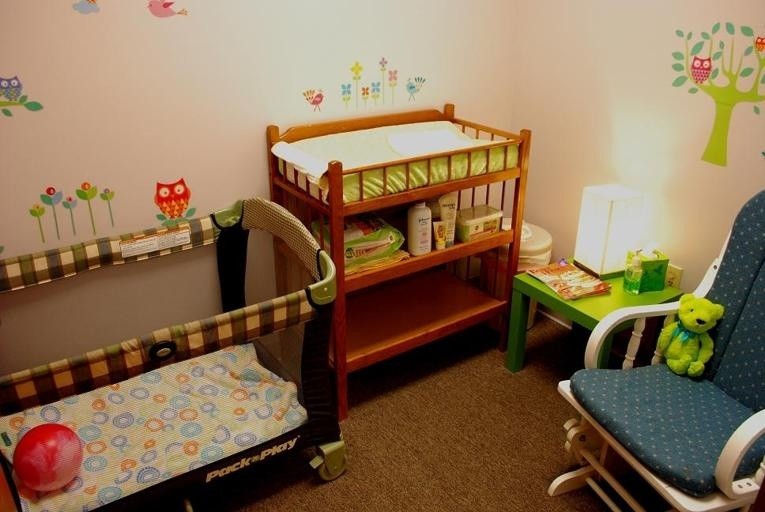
[1,194,351,512]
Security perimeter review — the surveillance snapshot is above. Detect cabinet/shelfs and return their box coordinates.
[266,102,534,420]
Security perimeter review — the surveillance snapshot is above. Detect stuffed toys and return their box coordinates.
[657,291,725,379]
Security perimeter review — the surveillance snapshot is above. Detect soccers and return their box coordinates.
[13,425,83,493]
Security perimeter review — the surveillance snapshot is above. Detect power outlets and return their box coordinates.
[665,264,684,290]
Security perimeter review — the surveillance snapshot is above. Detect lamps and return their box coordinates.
[570,183,643,280]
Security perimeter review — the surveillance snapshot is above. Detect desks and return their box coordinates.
[501,257,682,375]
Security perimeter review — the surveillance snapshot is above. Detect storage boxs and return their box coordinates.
[455,204,505,245]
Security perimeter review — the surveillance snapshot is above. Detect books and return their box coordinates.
[526,255,613,302]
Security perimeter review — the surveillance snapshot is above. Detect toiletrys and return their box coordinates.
[433,220,448,252]
[439,194,459,248]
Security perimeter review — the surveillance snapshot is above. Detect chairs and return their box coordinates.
[542,180,764,512]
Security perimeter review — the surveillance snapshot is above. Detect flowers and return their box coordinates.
[341,56,399,109]
